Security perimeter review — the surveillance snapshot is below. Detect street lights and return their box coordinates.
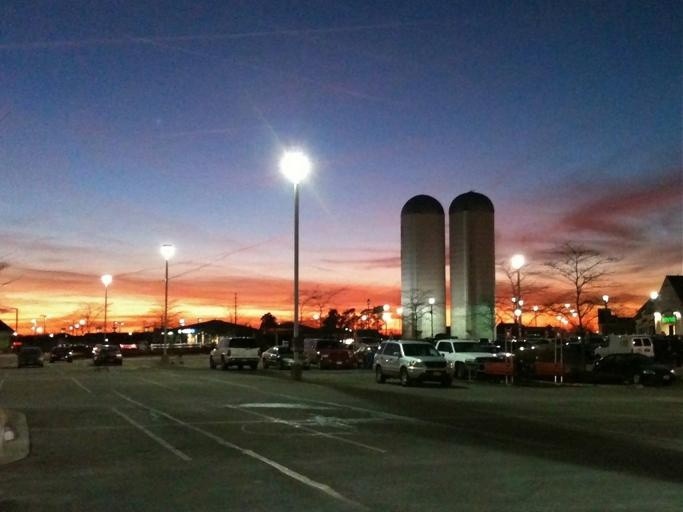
[160,242,176,361]
[510,255,525,337]
[31,313,86,336]
[533,294,609,327]
[311,297,442,340]
[101,274,112,338]
[280,150,310,378]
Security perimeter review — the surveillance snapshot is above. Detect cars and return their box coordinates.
[592,354,675,384]
[10,342,44,367]
[50,340,148,366]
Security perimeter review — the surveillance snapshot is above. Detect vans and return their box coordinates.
[592,334,655,358]
